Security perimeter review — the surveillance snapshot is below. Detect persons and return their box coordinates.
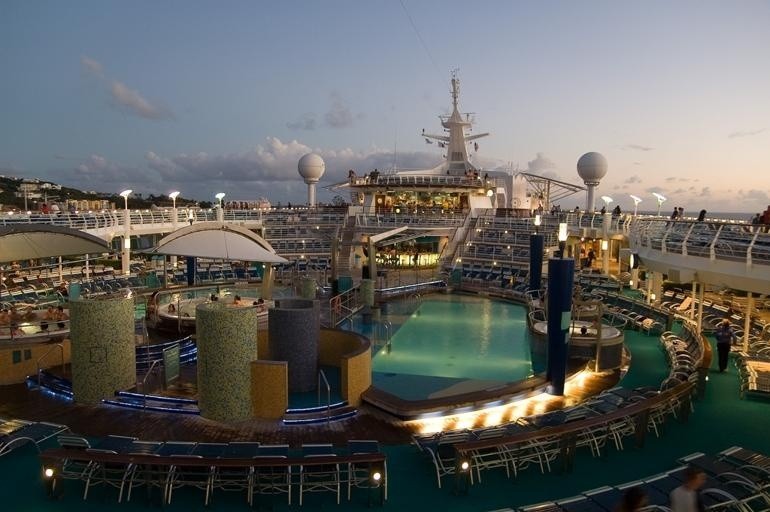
[607,483,655,511]
[668,466,709,512]
[611,205,620,231]
[697,209,708,222]
[550,205,556,215]
[574,205,582,218]
[677,208,686,218]
[221,200,225,208]
[714,318,737,375]
[573,324,595,338]
[277,201,282,208]
[70,209,78,220]
[259,298,264,305]
[233,201,235,210]
[670,206,677,218]
[235,298,243,305]
[245,202,248,209]
[760,206,769,232]
[252,302,257,306]
[750,213,759,224]
[600,205,606,216]
[537,204,544,211]
[588,248,595,268]
[233,202,238,209]
[226,202,231,209]
[211,296,218,303]
[287,201,291,209]
[181,312,190,317]
[185,206,197,225]
[40,203,49,213]
[0,260,69,338]
[555,205,560,211]
[231,295,238,305]
[166,303,178,313]
[365,167,490,270]
[50,202,61,217]
[759,210,766,225]
[210,294,214,302]
[239,201,243,211]
[347,170,356,184]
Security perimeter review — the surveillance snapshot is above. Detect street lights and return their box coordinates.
[215,193,225,208]
[630,195,642,216]
[169,191,180,208]
[120,190,132,211]
[558,222,569,258]
[602,196,613,213]
[534,215,541,235]
[653,193,666,217]
